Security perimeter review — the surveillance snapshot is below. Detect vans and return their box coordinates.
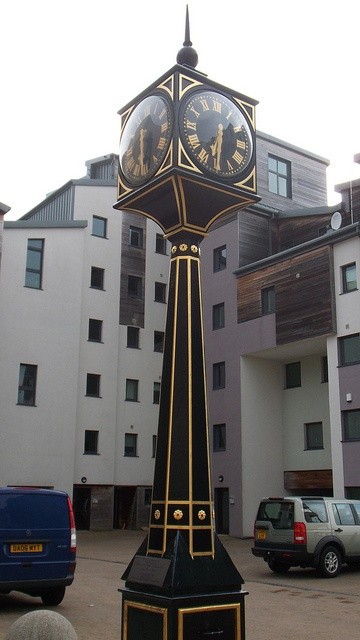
[0,484,78,608]
[252,495,360,578]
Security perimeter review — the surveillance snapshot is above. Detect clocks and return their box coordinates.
[181,89,255,184]
[118,93,173,189]
